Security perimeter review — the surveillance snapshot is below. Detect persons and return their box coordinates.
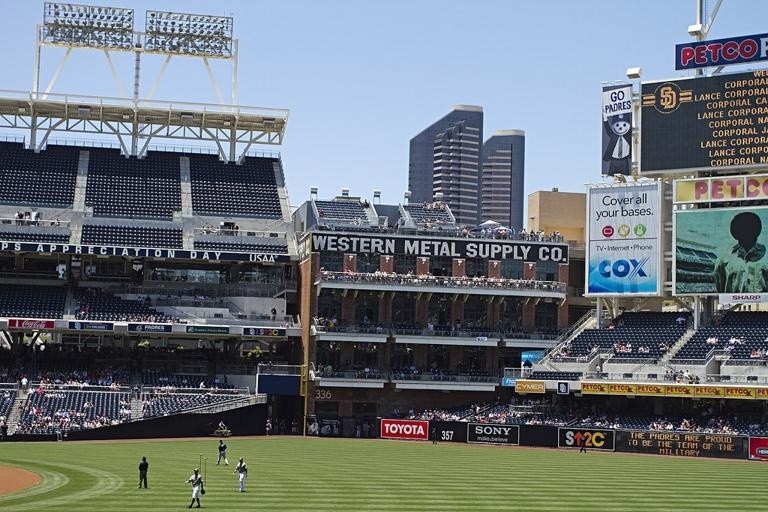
[184,468,206,508]
[0,199,767,453]
[136,456,149,489]
[232,458,247,492]
[216,440,229,465]
